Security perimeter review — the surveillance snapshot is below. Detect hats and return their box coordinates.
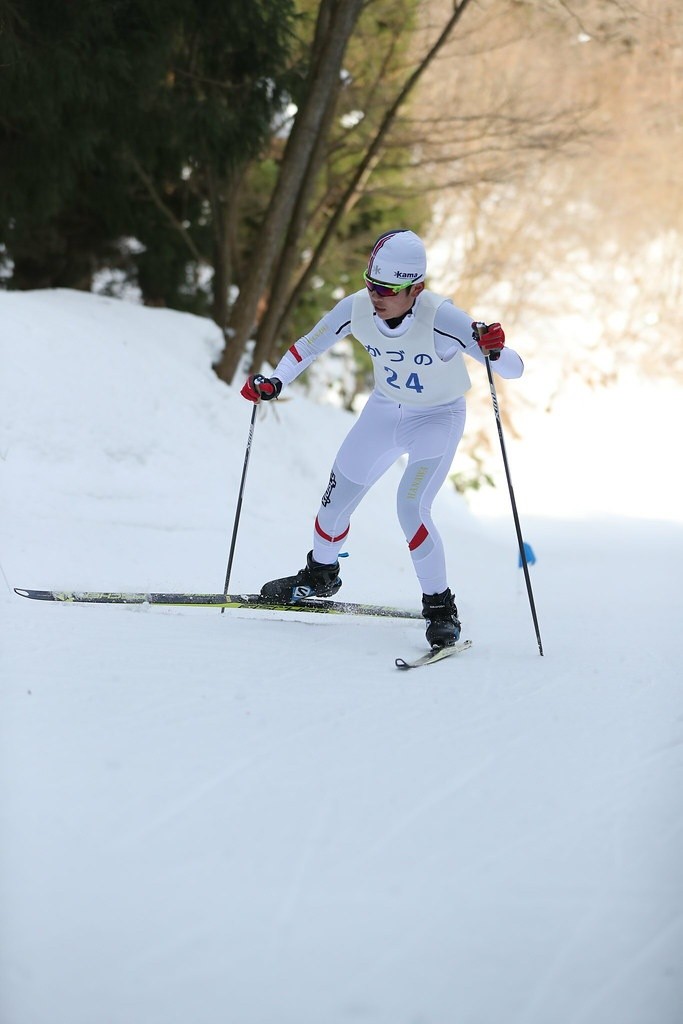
[368,229,427,285]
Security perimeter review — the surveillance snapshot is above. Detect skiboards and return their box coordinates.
[14,587,474,670]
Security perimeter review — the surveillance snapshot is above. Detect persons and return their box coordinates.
[242,229,523,647]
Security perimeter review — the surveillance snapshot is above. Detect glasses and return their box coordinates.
[363,270,423,297]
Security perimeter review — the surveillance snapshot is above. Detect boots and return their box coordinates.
[422,588,460,647]
[260,550,342,601]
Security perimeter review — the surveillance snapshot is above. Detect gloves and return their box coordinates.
[471,321,505,361]
[241,374,283,403]
